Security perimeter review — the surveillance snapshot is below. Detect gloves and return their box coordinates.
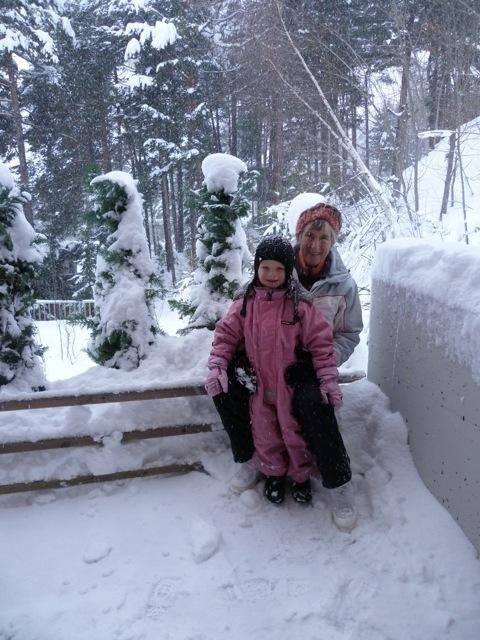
[204,355,229,396]
[318,368,344,408]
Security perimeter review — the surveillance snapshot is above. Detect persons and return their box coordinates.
[211,202,364,532]
[204,234,344,506]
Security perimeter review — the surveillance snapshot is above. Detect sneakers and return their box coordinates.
[329,481,356,529]
[288,476,311,503]
[264,475,284,504]
[230,452,264,494]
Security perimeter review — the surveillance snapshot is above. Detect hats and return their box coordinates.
[296,203,343,240]
[239,236,299,325]
